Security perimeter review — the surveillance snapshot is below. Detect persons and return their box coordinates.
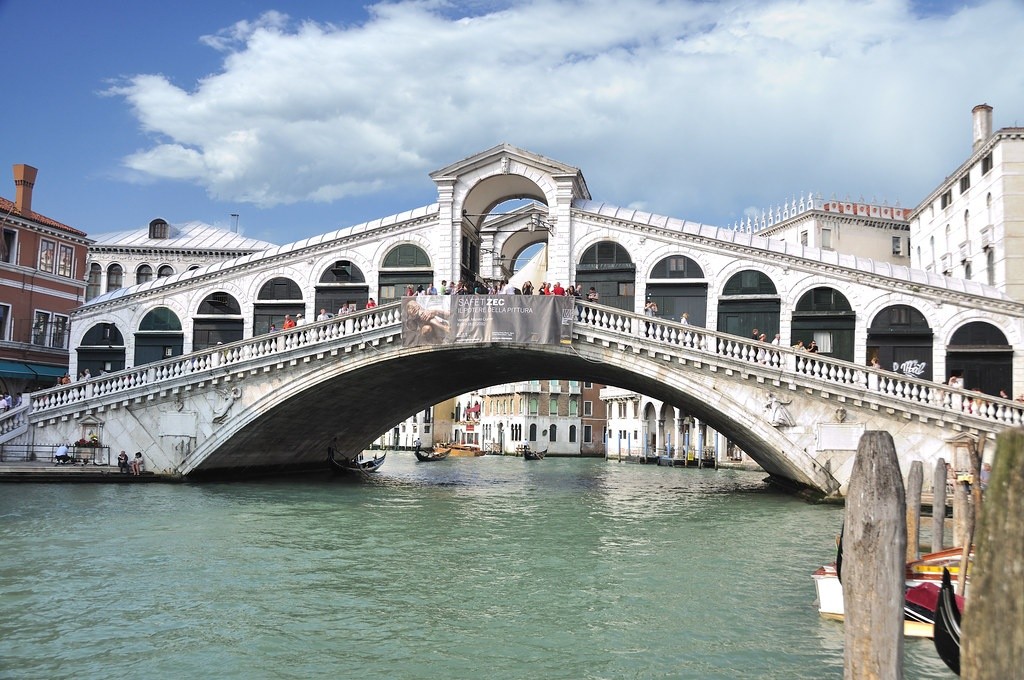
[414,438,421,453]
[338,298,377,333]
[131,452,143,475]
[945,463,957,491]
[0,367,131,416]
[644,301,658,339]
[763,394,796,427]
[681,313,689,335]
[283,315,294,347]
[405,280,534,296]
[980,463,992,491]
[55,445,69,464]
[118,450,130,475]
[212,387,242,423]
[941,375,1024,423]
[539,282,599,325]
[523,439,530,450]
[751,329,819,375]
[270,324,277,351]
[328,437,338,460]
[295,314,307,346]
[317,307,329,333]
[217,342,228,363]
[870,358,880,368]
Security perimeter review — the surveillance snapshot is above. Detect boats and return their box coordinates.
[524,449,548,460]
[813,540,972,638]
[330,447,389,472]
[415,447,452,461]
[431,443,487,457]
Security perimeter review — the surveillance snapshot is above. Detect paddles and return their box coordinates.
[334,448,370,477]
[413,443,441,460]
[529,447,544,461]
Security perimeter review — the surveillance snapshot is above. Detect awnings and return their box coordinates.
[0,359,72,380]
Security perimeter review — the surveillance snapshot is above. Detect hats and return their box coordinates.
[296,313,301,317]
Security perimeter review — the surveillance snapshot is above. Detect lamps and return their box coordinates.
[526,213,554,236]
